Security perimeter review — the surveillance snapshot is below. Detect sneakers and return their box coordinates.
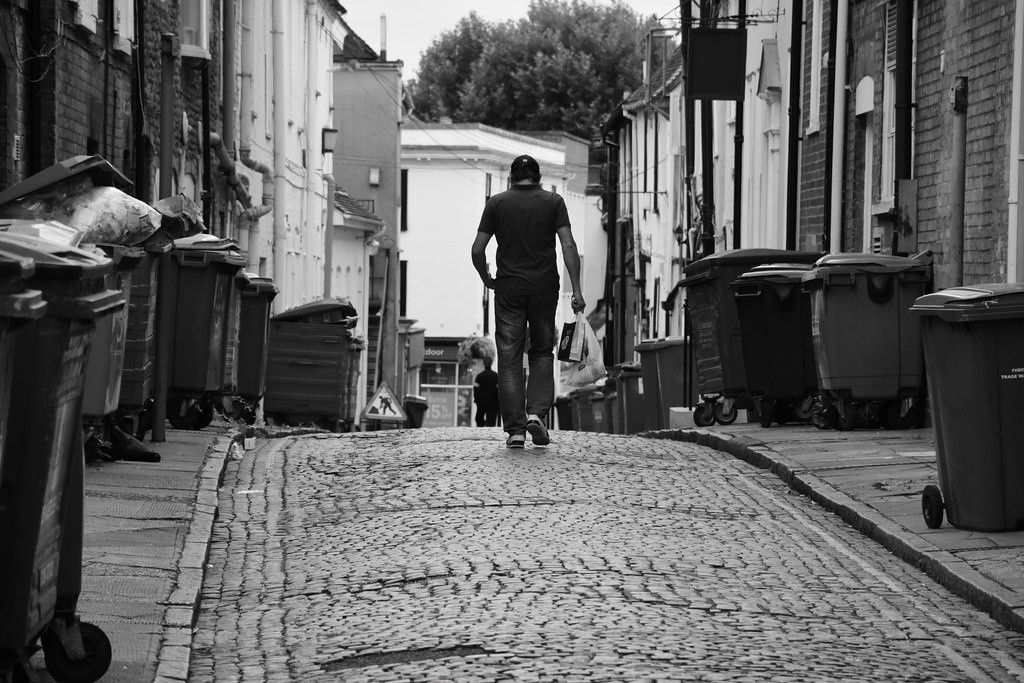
[527,414,550,445]
[506,434,525,448]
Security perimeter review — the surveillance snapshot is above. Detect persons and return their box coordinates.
[472,356,500,427]
[471,155,586,448]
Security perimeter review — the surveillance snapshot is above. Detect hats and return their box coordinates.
[510,155,539,173]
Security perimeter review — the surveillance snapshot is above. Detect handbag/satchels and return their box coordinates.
[558,312,585,362]
[565,311,607,387]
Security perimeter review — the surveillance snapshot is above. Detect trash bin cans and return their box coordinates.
[633,336,699,430]
[676,248,828,427]
[554,361,645,436]
[405,393,427,428]
[0,153,281,683]
[269,299,368,433]
[907,283,1024,533]
[799,248,933,432]
